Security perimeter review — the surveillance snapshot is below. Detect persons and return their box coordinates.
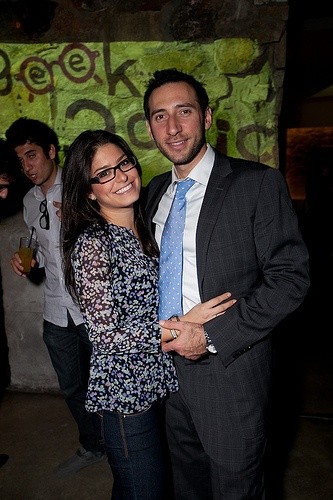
[60,130,238,500]
[140,67,310,500]
[0,117,107,478]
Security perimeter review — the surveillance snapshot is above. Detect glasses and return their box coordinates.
[39,200,49,230]
[89,155,136,184]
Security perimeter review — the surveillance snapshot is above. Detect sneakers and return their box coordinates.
[55,447,106,475]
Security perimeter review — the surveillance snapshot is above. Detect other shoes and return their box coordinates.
[0,453,9,467]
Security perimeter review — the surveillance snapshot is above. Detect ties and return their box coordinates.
[158,178,195,320]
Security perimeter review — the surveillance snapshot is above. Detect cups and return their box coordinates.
[19,237,37,272]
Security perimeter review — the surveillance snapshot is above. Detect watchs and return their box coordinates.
[204,328,218,354]
[167,315,180,339]
